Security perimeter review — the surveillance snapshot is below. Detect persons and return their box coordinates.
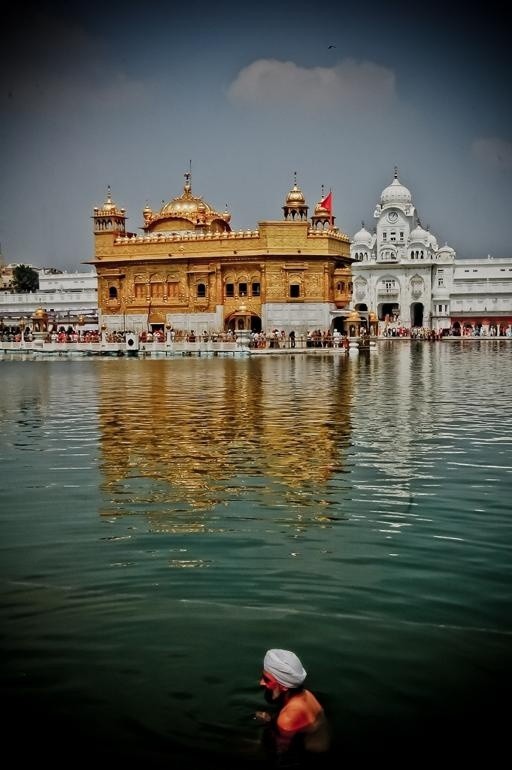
[189,329,236,342]
[255,647,329,758]
[249,329,267,347]
[352,327,369,346]
[106,331,132,343]
[45,329,100,342]
[306,329,349,347]
[269,329,295,347]
[382,317,511,338]
[1,326,32,341]
[140,329,188,343]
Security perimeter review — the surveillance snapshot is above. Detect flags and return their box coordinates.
[320,193,332,213]
